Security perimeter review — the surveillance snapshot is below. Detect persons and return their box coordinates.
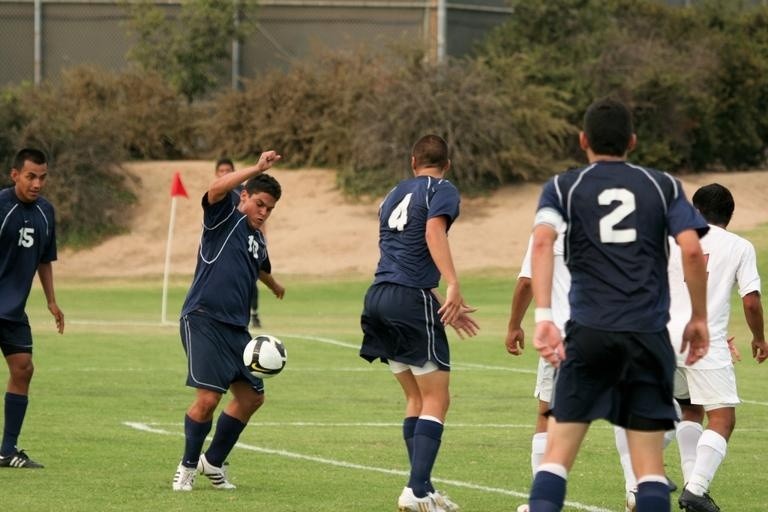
[172,151,285,492]
[216,159,266,328]
[528,98,709,512]
[0,148,64,468]
[506,165,637,511]
[669,184,768,511]
[358,135,480,510]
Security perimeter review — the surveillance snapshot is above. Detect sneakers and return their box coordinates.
[397,485,445,512]
[677,489,720,512]
[0,447,42,471]
[432,489,457,510]
[198,455,235,491]
[172,459,196,492]
[625,486,635,510]
[515,502,527,510]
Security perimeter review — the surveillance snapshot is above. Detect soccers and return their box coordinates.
[243,335,287,378]
